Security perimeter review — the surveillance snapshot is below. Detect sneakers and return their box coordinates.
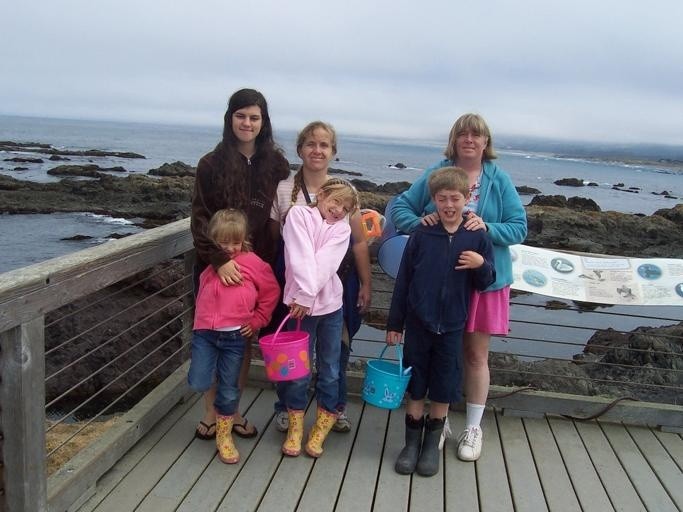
[458,424,482,461]
[438,417,452,449]
[331,412,351,432]
[277,410,290,433]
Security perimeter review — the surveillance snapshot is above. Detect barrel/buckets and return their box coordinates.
[258,315,311,381]
[363,343,411,408]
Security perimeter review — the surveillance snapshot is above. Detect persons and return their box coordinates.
[386,166,496,477]
[282,177,358,458]
[268,121,372,433]
[390,114,528,461]
[191,88,290,440]
[187,207,282,464]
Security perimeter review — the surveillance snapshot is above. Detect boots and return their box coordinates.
[305,406,340,459]
[395,414,446,476]
[216,412,240,464]
[282,409,304,457]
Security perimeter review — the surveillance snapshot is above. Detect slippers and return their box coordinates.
[233,418,258,439]
[197,420,217,441]
